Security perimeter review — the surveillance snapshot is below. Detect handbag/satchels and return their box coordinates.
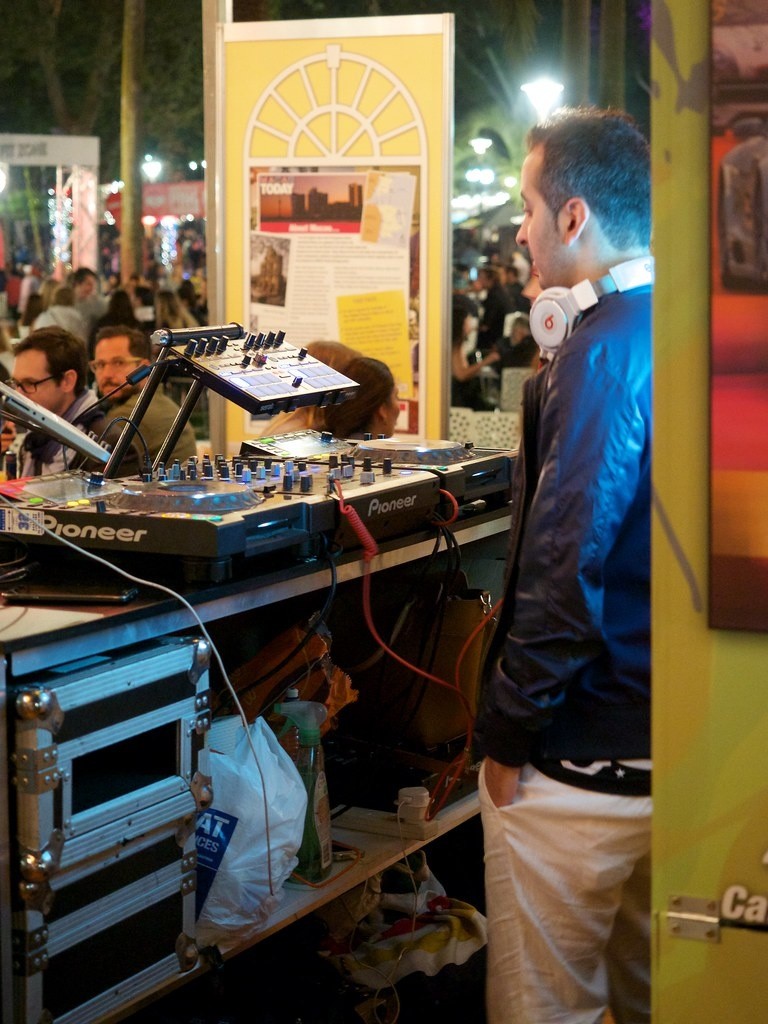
[193,715,308,934]
[338,581,489,752]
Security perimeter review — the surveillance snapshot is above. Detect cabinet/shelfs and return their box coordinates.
[1,508,512,1024]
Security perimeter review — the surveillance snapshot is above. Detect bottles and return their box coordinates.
[281,688,301,764]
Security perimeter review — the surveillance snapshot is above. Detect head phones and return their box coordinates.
[529,256,654,354]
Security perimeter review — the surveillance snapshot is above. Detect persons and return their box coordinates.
[478,110,656,1024]
[0,218,548,482]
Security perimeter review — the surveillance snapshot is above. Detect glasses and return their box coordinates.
[6,371,64,394]
[89,354,144,372]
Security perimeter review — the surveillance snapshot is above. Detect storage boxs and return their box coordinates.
[15,632,211,1023]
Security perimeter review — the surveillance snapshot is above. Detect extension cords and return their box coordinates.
[329,804,439,840]
